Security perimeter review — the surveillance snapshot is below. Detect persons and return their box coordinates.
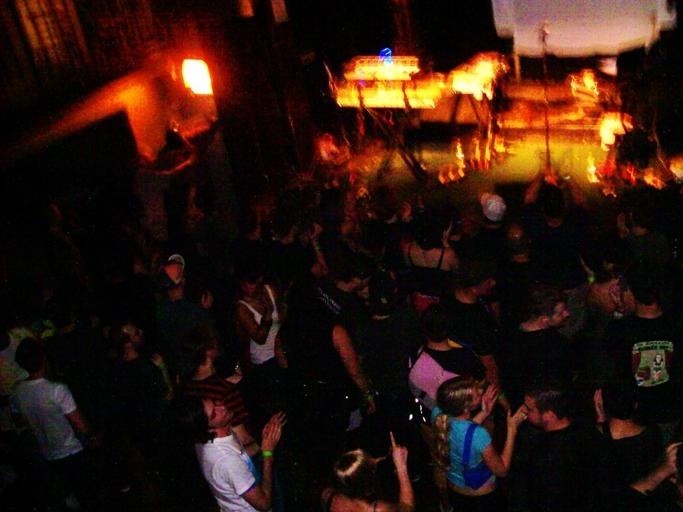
[0,129,683,512]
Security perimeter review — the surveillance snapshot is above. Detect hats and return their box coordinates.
[481,193,507,222]
